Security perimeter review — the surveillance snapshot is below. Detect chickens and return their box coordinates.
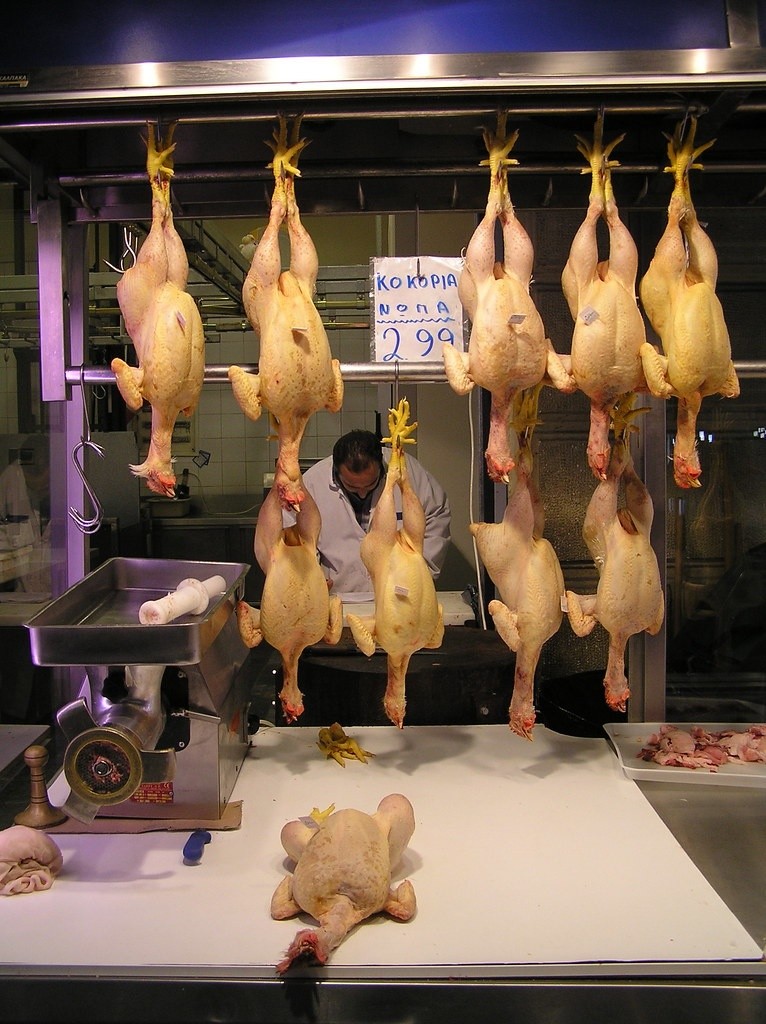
[109,114,741,978]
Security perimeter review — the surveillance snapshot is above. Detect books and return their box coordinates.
[338,592,375,603]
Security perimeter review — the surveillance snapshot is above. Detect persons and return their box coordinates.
[284,429,450,591]
